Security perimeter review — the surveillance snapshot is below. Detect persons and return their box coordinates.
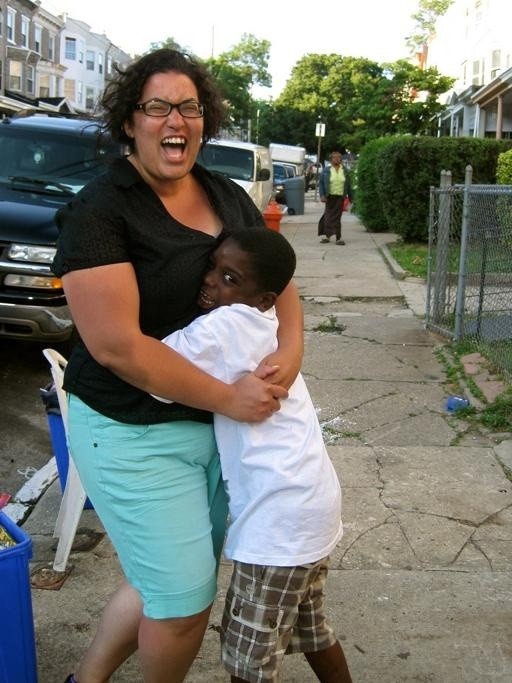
[53,48,304,683]
[318,152,353,245]
[148,228,353,683]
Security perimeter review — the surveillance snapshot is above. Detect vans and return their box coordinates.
[1,117,122,345]
[194,139,274,217]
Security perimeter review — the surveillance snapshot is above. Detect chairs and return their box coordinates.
[16,143,48,178]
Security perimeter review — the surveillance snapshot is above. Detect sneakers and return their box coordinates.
[320,239,329,243]
[336,240,344,245]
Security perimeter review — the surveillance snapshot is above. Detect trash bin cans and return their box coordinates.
[0,510,39,682]
[282,176,306,215]
[40,381,91,512]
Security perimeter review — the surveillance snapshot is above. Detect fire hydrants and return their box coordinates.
[262,198,282,233]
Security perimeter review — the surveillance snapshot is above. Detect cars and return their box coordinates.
[269,143,324,206]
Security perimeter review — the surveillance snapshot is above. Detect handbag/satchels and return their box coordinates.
[343,197,350,211]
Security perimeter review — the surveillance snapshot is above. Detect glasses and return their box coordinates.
[137,97,207,118]
